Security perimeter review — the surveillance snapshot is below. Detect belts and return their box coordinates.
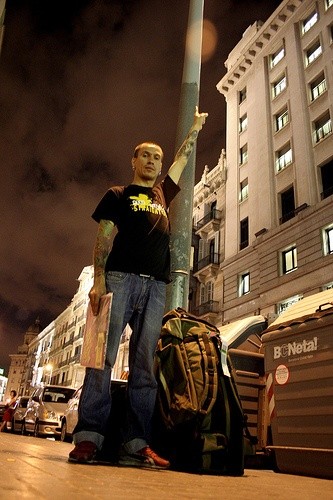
[131,272,159,281]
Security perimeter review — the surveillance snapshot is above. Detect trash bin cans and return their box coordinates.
[214,314,272,472]
[261,287,333,480]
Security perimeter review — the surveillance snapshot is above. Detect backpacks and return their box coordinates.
[153,305,258,475]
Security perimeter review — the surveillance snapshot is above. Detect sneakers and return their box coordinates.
[69,440,97,466]
[115,447,171,469]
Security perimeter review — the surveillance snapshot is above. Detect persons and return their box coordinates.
[0,390,16,431]
[69,105,208,469]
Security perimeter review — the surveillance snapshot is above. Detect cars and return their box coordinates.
[0,382,77,440]
[59,378,128,443]
